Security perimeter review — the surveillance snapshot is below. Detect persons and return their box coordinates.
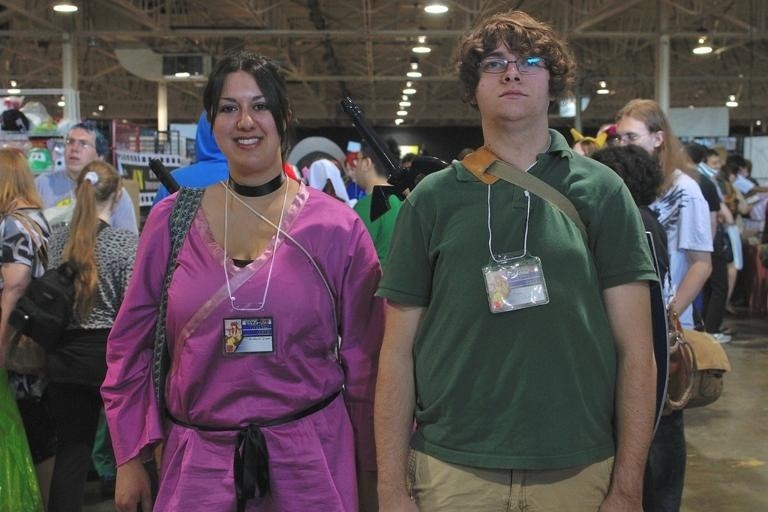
[157,112,228,204]
[295,137,475,275]
[101,52,384,511]
[574,99,768,512]
[0,122,139,512]
[373,10,662,511]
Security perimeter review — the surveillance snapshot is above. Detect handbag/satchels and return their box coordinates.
[50,328,109,396]
[7,260,79,353]
[662,331,731,416]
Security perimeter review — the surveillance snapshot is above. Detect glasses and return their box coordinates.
[65,137,95,149]
[612,128,663,143]
[479,57,547,75]
